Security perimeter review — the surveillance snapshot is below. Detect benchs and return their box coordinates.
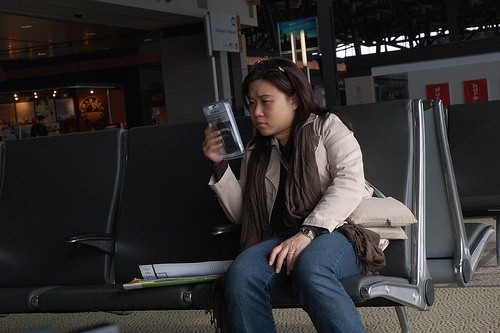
[445,101,500,266]
[0,97,435,333]
[422,100,495,287]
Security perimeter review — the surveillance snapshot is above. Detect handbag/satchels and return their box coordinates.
[345,196,418,239]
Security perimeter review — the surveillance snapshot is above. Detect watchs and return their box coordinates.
[300,226,314,242]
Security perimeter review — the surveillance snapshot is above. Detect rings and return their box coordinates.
[287,252,294,255]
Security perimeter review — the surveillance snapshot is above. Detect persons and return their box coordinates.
[31,116,48,137]
[202,59,389,333]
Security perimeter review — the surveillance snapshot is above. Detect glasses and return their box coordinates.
[250,60,285,73]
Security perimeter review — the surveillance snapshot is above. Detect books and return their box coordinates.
[123,274,223,290]
